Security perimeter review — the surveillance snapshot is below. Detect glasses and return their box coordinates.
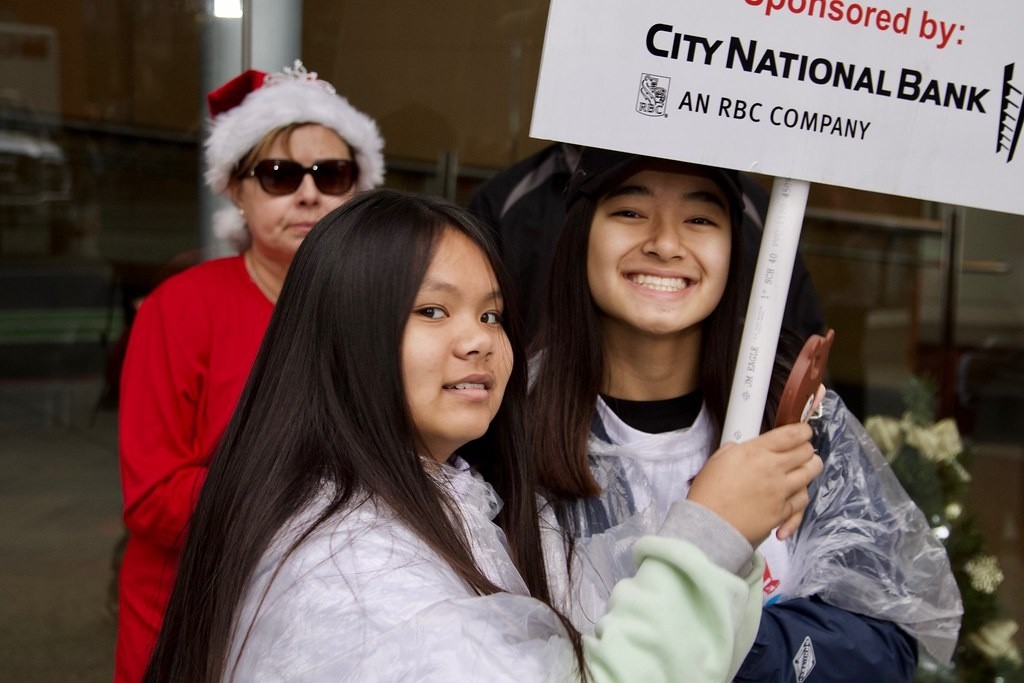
[239,159,360,195]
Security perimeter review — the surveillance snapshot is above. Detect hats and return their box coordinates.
[203,59,385,251]
[563,147,746,215]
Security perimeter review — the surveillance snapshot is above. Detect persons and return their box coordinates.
[142,188,824,683]
[118,69,386,683]
[464,148,963,682]
